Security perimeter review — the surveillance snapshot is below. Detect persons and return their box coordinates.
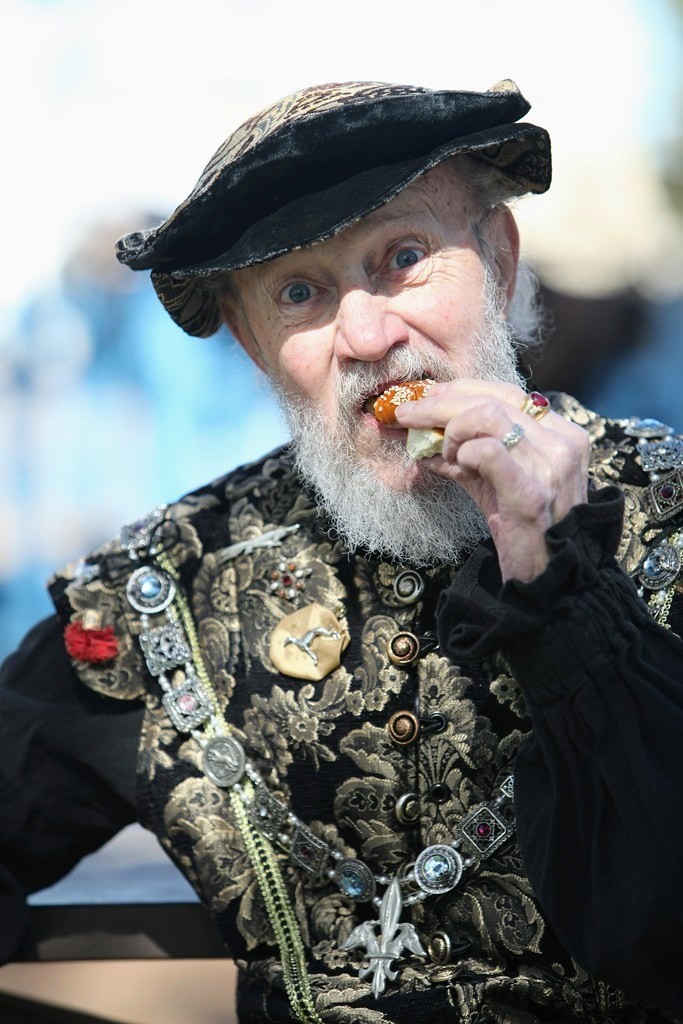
[1,74,683,1024]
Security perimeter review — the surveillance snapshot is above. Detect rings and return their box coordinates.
[522,388,552,423]
[503,423,531,449]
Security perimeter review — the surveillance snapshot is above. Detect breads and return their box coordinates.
[405,425,447,460]
[368,378,440,429]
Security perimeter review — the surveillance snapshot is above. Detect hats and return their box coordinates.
[114,79,553,339]
